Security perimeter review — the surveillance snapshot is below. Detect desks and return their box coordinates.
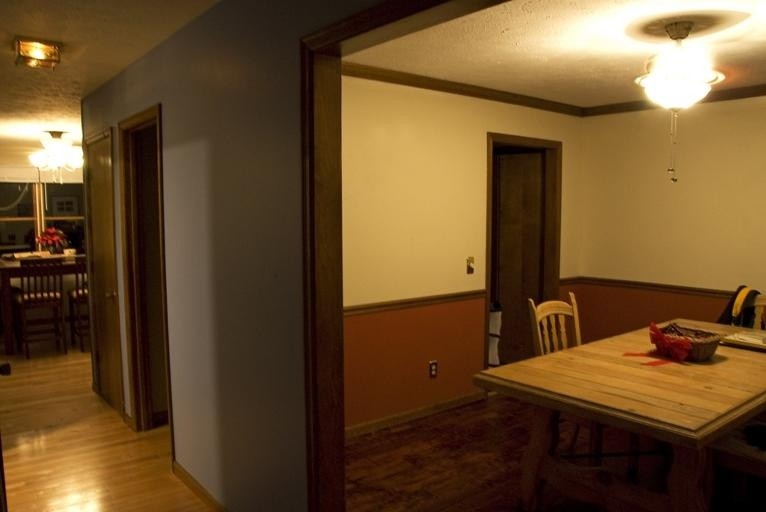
[0,252,90,355]
[472,318,766,512]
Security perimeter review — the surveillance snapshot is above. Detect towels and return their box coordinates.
[717,285,761,328]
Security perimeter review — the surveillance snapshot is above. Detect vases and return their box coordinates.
[50,246,64,254]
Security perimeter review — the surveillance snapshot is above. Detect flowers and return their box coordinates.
[35,227,69,254]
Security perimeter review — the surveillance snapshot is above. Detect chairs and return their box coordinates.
[527,292,642,466]
[13,257,68,359]
[729,294,766,331]
[67,255,91,353]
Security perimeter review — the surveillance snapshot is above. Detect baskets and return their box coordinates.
[649,322,721,362]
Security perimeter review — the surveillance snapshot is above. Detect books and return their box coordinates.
[723,331,766,351]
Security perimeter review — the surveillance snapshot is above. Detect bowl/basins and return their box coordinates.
[63,248,77,255]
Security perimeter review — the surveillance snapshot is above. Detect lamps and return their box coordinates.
[11,38,62,71]
[634,19,729,181]
[28,131,84,186]
